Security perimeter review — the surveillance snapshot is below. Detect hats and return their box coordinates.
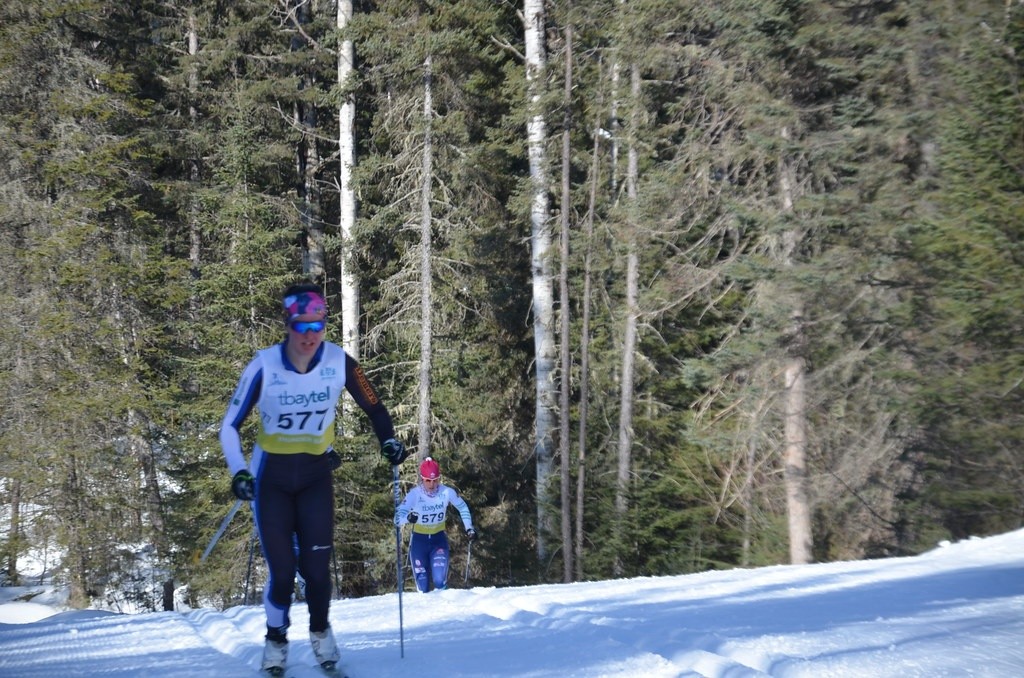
[419,457,441,480]
[284,292,326,323]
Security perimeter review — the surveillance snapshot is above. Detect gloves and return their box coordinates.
[380,440,408,465]
[467,529,477,540]
[231,469,256,502]
[407,512,419,524]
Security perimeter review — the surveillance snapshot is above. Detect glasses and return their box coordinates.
[286,318,328,334]
[423,479,438,483]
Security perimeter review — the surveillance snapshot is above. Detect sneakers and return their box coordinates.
[309,623,340,668]
[262,634,289,677]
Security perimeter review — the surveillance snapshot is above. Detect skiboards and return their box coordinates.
[259,663,349,678]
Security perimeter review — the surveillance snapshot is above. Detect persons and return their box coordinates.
[220,286,409,678]
[290,533,305,605]
[394,457,477,593]
